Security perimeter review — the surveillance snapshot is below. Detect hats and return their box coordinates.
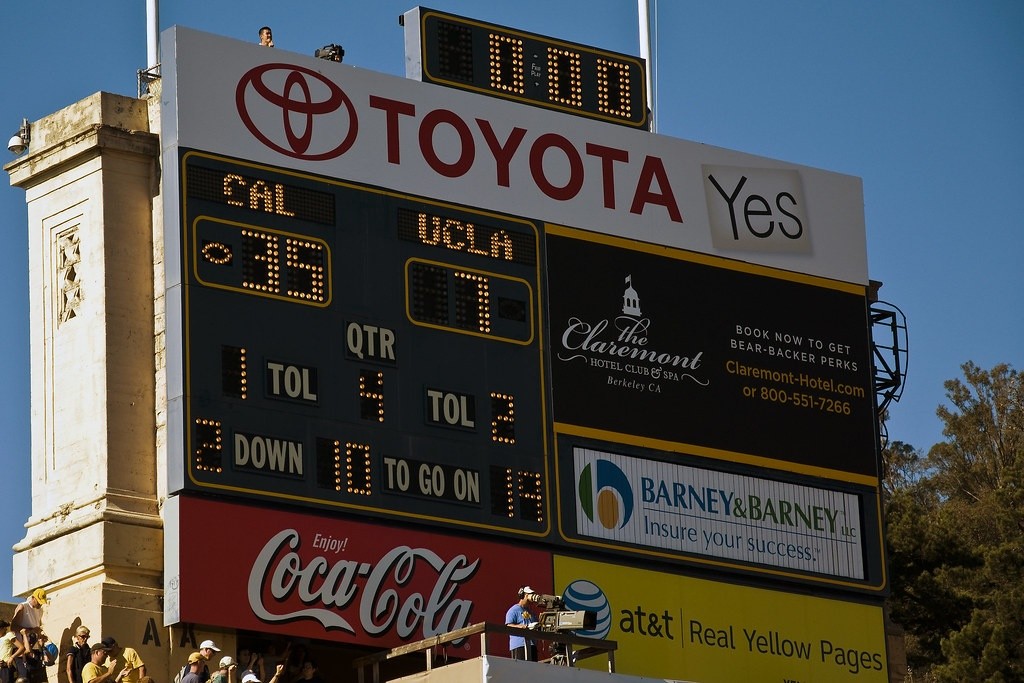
[218,655,238,668]
[524,586,534,593]
[199,640,222,652]
[101,636,116,647]
[31,588,47,605]
[90,643,112,651]
[188,651,209,665]
[242,674,262,683]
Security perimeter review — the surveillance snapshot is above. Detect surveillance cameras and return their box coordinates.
[8,135,27,155]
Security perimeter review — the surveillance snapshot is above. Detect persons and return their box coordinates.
[16,676,30,683]
[180,652,208,683]
[258,26,275,47]
[66,625,92,683]
[81,641,134,683]
[237,641,326,683]
[0,619,26,683]
[10,588,49,683]
[504,585,538,661]
[102,637,146,683]
[211,655,238,683]
[137,676,154,683]
[13,627,42,683]
[184,639,222,683]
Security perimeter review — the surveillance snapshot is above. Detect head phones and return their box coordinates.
[518,586,526,600]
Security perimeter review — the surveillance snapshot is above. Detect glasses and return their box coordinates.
[244,653,250,656]
[78,635,90,639]
